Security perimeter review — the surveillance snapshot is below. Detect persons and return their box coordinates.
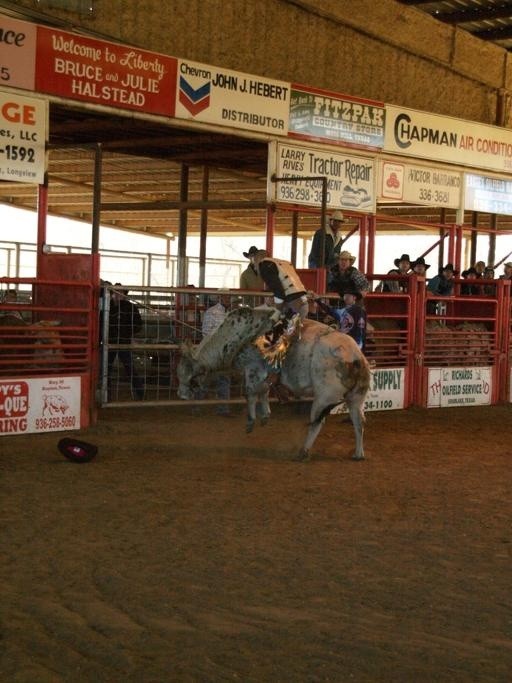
[309,283,367,355]
[201,287,235,341]
[308,209,345,290]
[99,283,149,400]
[0,287,24,321]
[326,249,511,328]
[239,245,267,306]
[250,248,310,322]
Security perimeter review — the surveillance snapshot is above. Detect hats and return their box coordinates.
[243,247,257,258]
[110,283,128,295]
[339,288,362,298]
[394,254,512,275]
[333,251,355,264]
[328,211,349,223]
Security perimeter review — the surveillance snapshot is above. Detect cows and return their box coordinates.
[168,306,371,462]
[324,318,490,368]
[1,314,65,376]
